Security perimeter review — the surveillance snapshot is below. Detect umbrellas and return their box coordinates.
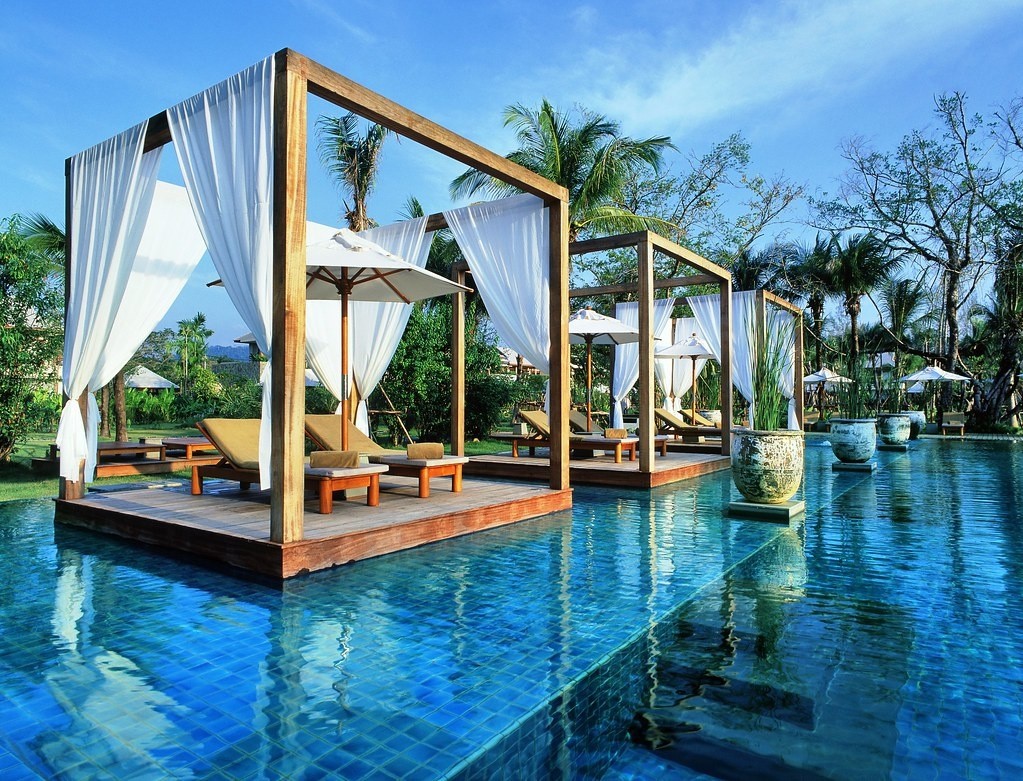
[804,367,855,420]
[208,227,473,452]
[655,333,716,426]
[893,365,971,423]
[569,307,662,430]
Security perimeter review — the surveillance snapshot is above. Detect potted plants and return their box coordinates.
[901,373,945,440]
[878,355,911,446]
[730,296,824,503]
[830,319,877,462]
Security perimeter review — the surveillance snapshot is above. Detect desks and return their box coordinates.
[97,442,167,465]
[162,439,218,459]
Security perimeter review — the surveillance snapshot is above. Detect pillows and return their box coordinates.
[742,421,749,426]
[604,428,627,439]
[310,451,359,468]
[407,443,444,460]
[714,421,734,428]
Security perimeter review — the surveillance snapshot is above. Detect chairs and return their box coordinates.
[677,409,720,427]
[569,410,666,457]
[192,418,390,514]
[942,412,964,436]
[654,409,729,440]
[304,414,469,498]
[513,411,639,463]
